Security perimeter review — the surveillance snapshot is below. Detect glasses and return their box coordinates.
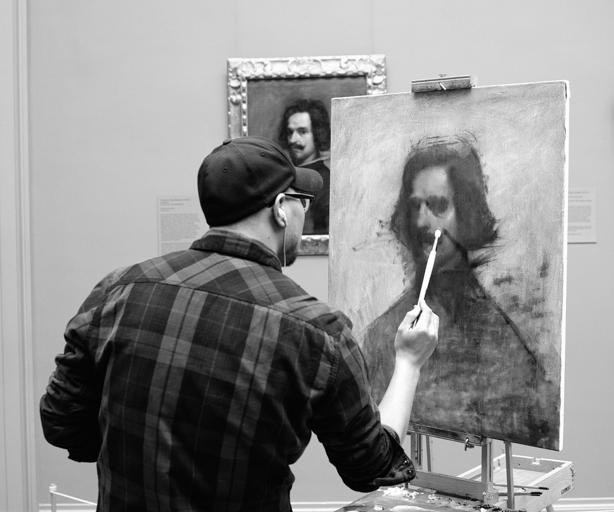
[267,190,315,213]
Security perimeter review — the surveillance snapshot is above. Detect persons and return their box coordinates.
[274,100,330,238]
[35,136,441,511]
[355,134,558,449]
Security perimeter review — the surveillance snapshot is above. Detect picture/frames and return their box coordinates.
[226,54,386,256]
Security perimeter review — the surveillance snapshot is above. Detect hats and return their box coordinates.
[197,134,325,228]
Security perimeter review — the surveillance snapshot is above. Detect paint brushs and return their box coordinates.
[495,484,549,496]
[411,229,442,329]
